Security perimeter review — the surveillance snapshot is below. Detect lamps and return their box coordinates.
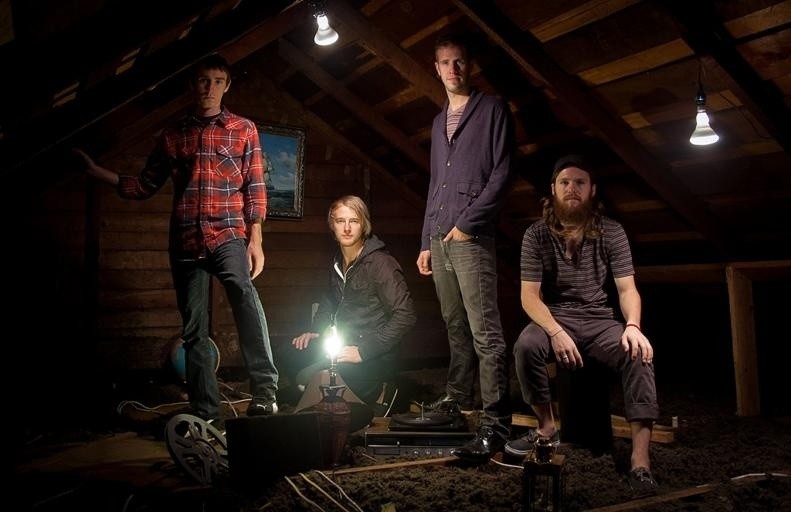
[314,3,340,46]
[317,326,347,401]
[689,86,720,146]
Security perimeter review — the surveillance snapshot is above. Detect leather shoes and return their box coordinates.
[426,394,460,416]
[452,427,503,458]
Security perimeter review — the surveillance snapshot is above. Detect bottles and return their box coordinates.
[523,436,566,512]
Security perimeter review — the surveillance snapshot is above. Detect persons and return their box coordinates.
[73,54,280,433]
[279,194,421,420]
[504,156,660,492]
[410,34,516,456]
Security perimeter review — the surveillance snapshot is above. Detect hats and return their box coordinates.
[553,155,586,171]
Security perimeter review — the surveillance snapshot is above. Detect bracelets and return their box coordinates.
[625,322,643,330]
[550,328,563,340]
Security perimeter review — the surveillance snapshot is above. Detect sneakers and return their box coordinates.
[631,466,657,486]
[183,418,226,441]
[246,399,278,415]
[504,430,559,455]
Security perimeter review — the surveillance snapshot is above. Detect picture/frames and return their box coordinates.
[248,119,307,221]
